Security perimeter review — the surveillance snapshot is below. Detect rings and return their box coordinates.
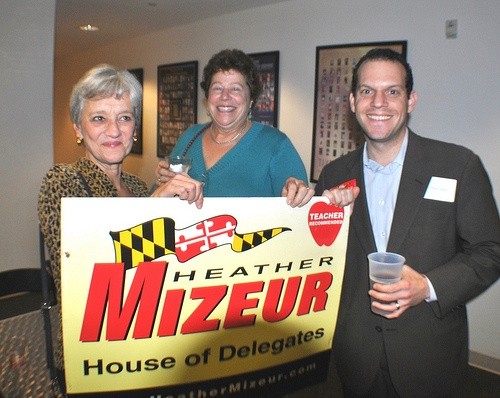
[395,301,401,311]
[306,187,310,190]
[158,175,160,182]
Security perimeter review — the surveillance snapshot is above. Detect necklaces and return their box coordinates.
[208,118,248,145]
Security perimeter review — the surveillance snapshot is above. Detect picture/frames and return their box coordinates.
[126,68,145,153]
[156,60,202,158]
[244,50,280,129]
[309,40,408,184]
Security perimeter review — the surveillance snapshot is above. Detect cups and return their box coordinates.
[164,154,192,176]
[368,252,407,316]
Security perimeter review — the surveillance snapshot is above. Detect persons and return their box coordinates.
[316,47,500,398]
[150,46,316,208]
[37,64,204,398]
